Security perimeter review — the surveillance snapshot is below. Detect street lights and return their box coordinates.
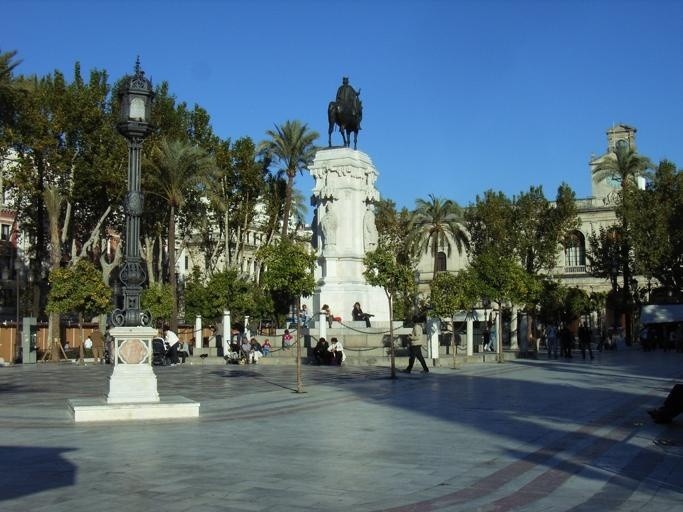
[108,54,152,326]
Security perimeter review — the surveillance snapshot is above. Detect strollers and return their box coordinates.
[152,337,171,367]
[225,339,248,365]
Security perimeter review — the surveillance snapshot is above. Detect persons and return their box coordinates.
[84,336,93,348]
[646,383,683,424]
[64,341,73,352]
[336,76,361,135]
[482,324,497,352]
[163,326,190,364]
[313,338,334,366]
[322,305,333,328]
[352,302,371,328]
[544,320,620,360]
[300,305,309,328]
[282,329,293,351]
[639,324,683,353]
[399,316,429,374]
[326,338,346,367]
[237,322,274,365]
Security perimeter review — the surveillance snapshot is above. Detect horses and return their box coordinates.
[327,88,363,150]
